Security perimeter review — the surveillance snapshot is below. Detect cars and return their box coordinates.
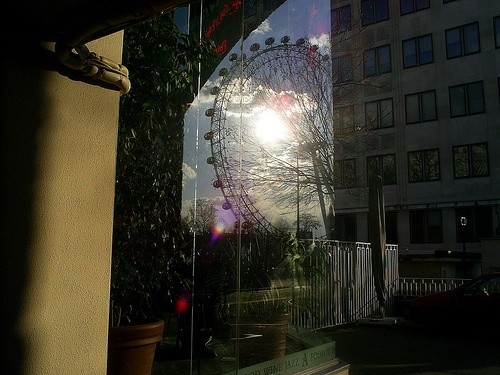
[411,271,500,344]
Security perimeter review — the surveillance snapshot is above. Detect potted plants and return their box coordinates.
[106,10,225,374]
[207,228,333,367]
